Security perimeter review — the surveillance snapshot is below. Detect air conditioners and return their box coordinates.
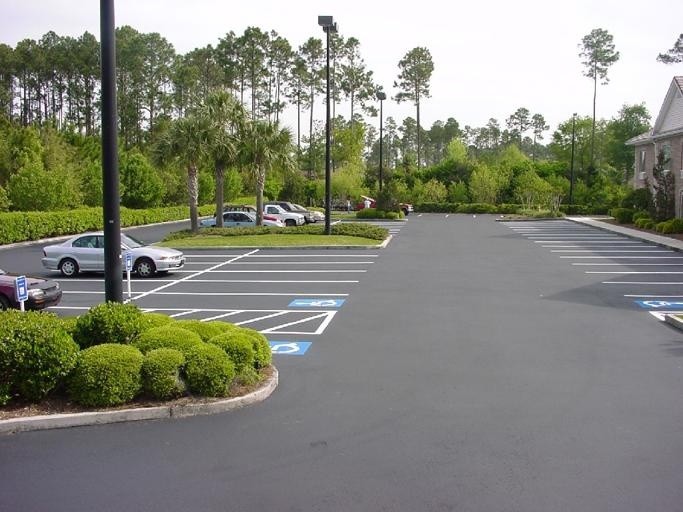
[639,172,647,180]
[663,170,670,176]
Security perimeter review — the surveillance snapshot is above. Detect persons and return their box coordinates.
[363,198,371,208]
[346,197,354,215]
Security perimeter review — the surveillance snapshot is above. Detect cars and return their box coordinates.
[356,195,413,214]
[40,229,185,278]
[196,201,323,232]
[0,264,61,316]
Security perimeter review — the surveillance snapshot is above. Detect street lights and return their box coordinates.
[376,91,386,193]
[317,16,339,235]
[567,113,577,215]
